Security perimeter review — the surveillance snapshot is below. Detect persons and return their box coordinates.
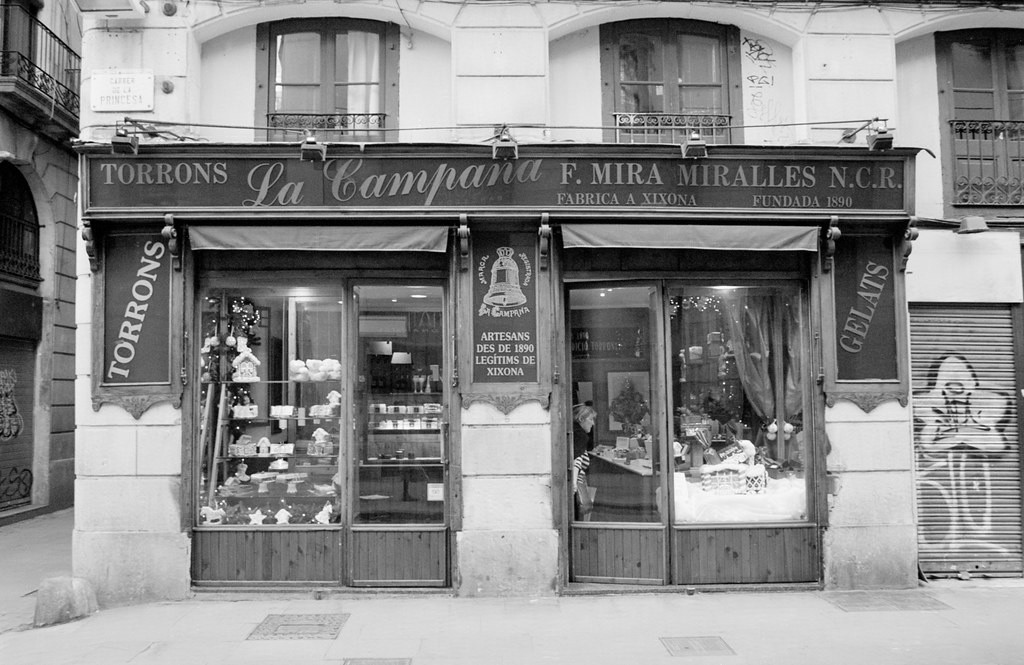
[573,405,598,522]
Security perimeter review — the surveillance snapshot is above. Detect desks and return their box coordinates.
[587,438,660,521]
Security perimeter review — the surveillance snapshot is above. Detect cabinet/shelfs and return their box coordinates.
[679,301,744,420]
[195,375,347,527]
[362,397,447,498]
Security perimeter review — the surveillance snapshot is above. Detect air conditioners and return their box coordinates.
[359,315,409,337]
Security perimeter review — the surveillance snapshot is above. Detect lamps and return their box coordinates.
[842,116,893,151]
[299,127,328,162]
[111,116,159,157]
[491,123,518,160]
[680,132,709,158]
